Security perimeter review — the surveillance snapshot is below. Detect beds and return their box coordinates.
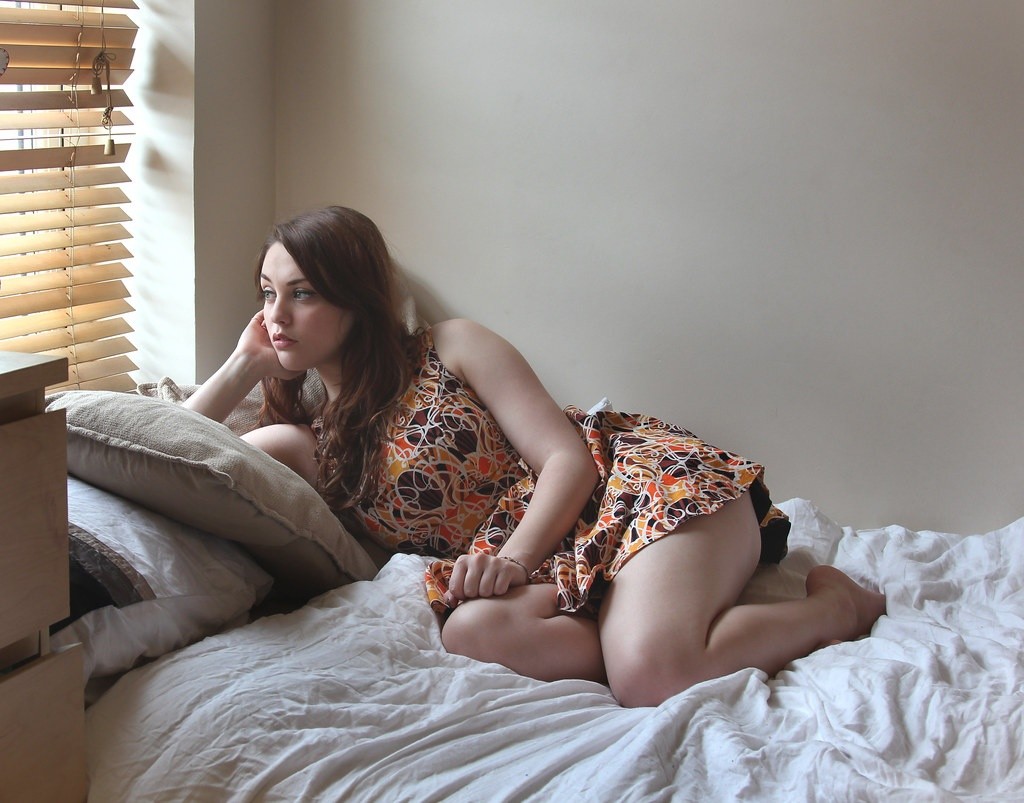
[89,499,1024,803]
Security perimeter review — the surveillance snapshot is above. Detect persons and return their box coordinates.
[179,204,886,708]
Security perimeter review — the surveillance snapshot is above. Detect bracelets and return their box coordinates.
[498,556,529,585]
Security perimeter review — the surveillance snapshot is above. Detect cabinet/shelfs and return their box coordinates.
[0,351,89,803]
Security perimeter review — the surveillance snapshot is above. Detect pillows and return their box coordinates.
[41,295,430,679]
